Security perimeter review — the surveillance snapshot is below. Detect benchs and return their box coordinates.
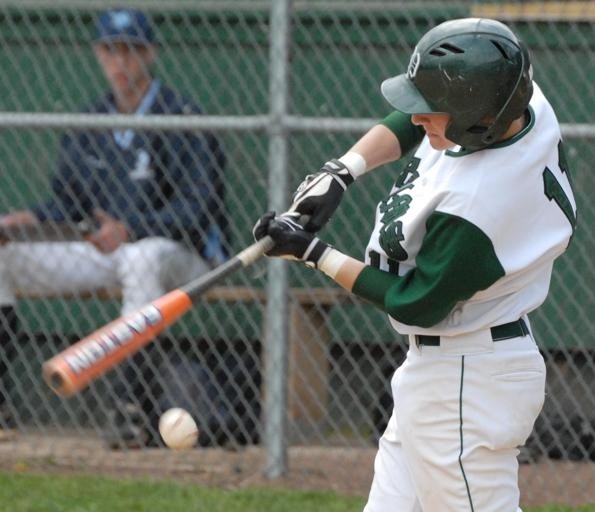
[0,22,595,448]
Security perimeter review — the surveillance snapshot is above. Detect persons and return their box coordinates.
[251,17,580,512]
[0,6,234,450]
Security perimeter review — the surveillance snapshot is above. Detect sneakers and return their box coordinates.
[109,404,149,448]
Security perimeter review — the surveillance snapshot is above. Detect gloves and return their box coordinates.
[252,210,332,270]
[289,158,355,234]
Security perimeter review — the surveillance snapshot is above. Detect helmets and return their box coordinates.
[381,18,533,151]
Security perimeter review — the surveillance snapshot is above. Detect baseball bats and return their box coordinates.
[42,210,310,399]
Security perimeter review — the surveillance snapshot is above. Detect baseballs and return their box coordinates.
[159,406,200,448]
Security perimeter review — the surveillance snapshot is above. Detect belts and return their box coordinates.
[415,319,529,349]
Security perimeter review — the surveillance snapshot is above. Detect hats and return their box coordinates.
[95,9,151,46]
